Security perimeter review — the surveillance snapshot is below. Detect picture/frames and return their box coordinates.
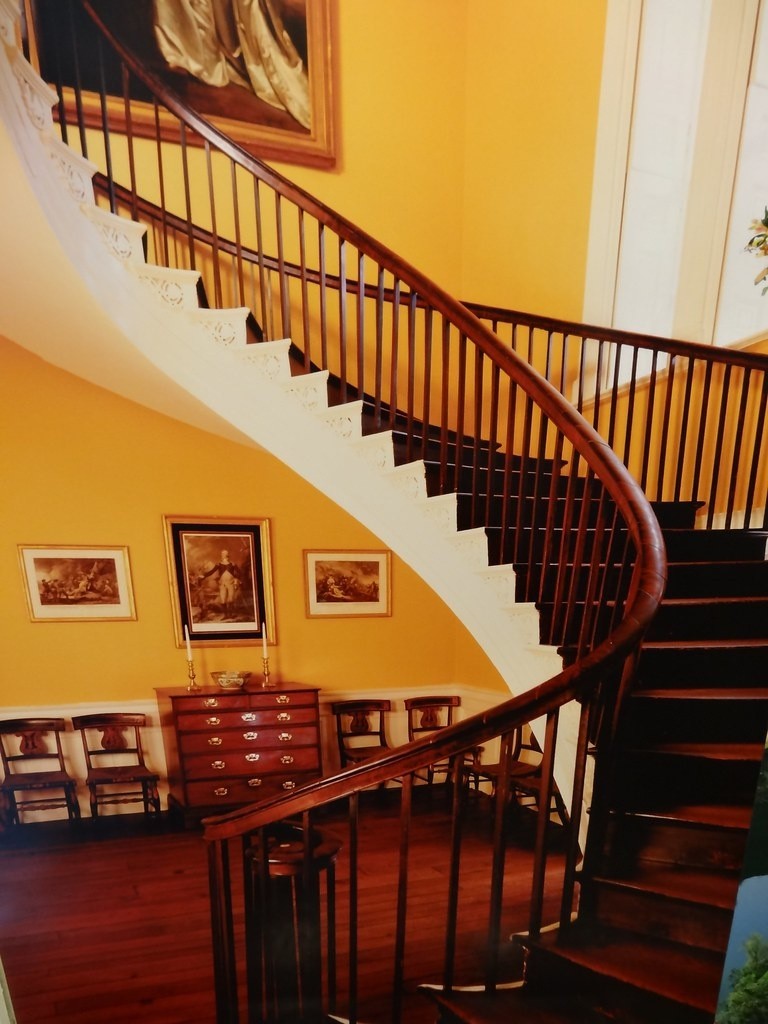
[16,0,344,174]
[15,542,138,623]
[302,548,393,618]
[159,512,280,647]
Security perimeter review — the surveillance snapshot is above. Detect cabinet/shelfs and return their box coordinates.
[152,679,324,836]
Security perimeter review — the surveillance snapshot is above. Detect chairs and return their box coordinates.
[321,699,414,814]
[507,748,564,839]
[0,718,81,834]
[69,712,163,831]
[402,696,485,804]
[458,723,545,822]
